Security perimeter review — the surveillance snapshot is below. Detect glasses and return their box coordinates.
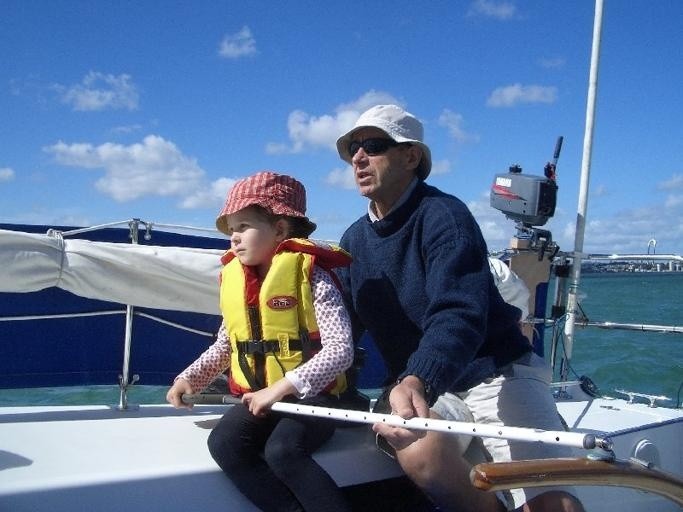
[345,137,394,159]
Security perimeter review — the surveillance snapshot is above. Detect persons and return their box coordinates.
[166,172,355,512]
[332,104,586,512]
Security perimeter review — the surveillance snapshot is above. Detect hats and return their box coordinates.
[335,103,432,184]
[215,172,317,235]
